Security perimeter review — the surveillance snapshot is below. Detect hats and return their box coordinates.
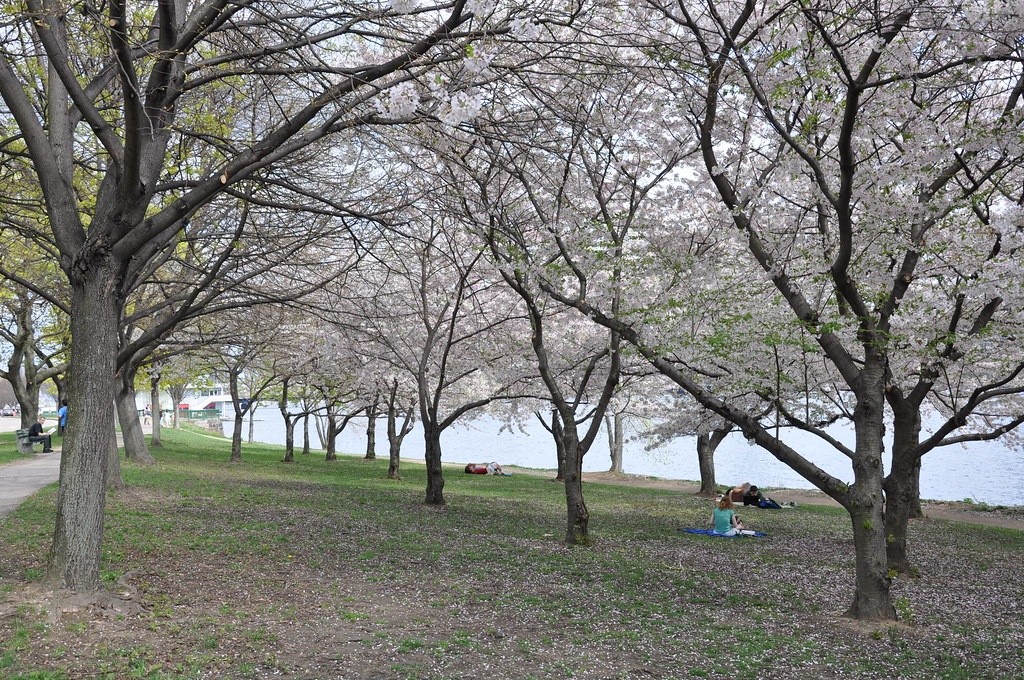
[750,486,758,491]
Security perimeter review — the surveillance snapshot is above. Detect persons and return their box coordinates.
[727,482,782,510]
[465,462,511,477]
[160,408,172,428]
[59,401,69,434]
[711,498,743,536]
[28,415,55,453]
[144,404,153,425]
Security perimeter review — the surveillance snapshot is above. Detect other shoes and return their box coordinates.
[43,449,53,453]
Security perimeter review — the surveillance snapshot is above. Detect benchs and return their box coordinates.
[16,429,43,453]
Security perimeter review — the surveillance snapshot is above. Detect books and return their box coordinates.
[48,427,57,435]
